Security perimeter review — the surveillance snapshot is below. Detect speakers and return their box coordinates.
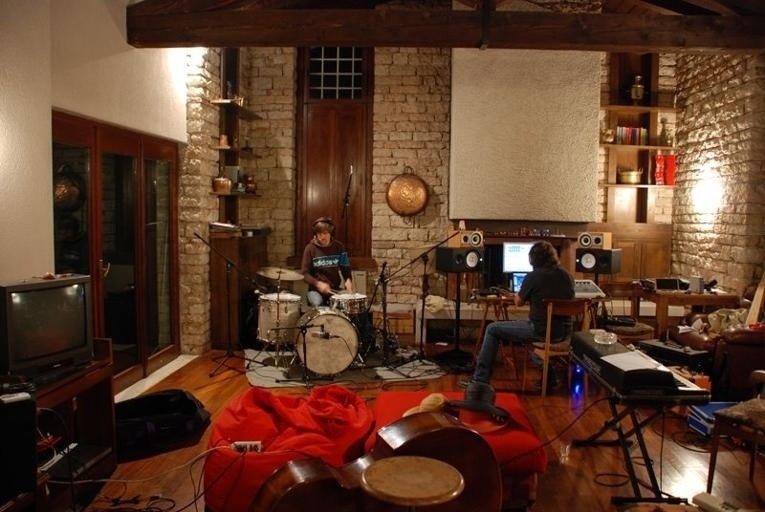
[458,229,486,247]
[434,245,486,274]
[577,230,613,250]
[574,247,624,275]
[0,396,41,506]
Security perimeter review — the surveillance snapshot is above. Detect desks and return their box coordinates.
[634,275,737,339]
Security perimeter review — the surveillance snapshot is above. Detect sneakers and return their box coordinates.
[531,376,559,390]
[457,378,476,388]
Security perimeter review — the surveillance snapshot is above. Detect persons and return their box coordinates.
[300,216,381,353]
[457,239,577,390]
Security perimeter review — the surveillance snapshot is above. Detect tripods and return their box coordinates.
[209,263,268,377]
[246,276,299,373]
[362,278,408,370]
[393,252,440,368]
[275,330,335,393]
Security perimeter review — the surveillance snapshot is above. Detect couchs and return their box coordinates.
[665,286,765,403]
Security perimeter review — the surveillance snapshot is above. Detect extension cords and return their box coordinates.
[230,438,265,453]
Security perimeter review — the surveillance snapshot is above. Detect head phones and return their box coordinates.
[310,219,337,242]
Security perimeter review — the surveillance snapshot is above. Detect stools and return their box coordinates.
[366,392,549,511]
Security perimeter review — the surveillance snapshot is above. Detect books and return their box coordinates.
[615,125,649,147]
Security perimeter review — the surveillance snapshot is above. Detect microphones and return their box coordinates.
[311,332,331,340]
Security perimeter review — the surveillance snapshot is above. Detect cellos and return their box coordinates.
[249,410,505,512]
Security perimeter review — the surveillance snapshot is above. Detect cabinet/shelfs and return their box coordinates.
[210,227,272,349]
[478,234,579,275]
[0,337,116,512]
[212,47,262,229]
[612,239,670,284]
[598,50,677,221]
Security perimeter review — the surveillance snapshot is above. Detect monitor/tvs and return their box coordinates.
[499,241,535,274]
[511,272,528,293]
[0,272,95,385]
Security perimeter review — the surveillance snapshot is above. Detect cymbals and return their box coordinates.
[256,267,305,280]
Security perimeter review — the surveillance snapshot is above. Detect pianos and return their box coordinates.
[571,332,710,405]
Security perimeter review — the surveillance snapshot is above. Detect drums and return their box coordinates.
[258,293,302,345]
[341,292,367,315]
[330,294,341,309]
[295,307,358,376]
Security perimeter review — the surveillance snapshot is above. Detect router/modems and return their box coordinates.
[2,391,33,405]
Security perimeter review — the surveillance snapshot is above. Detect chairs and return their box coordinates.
[706,370,765,495]
[600,280,655,343]
[521,298,591,405]
